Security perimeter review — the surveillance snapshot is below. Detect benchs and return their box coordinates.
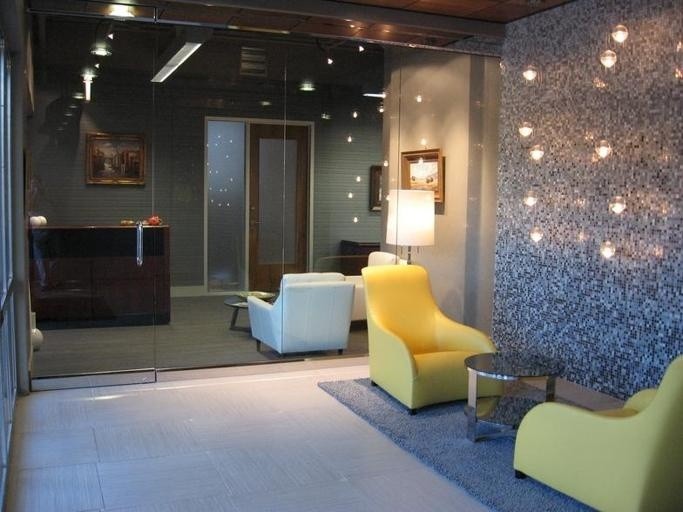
[520,376,625,411]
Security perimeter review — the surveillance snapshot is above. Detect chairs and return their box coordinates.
[247,273,355,359]
[362,265,497,414]
[344,251,407,333]
[514,355,683,512]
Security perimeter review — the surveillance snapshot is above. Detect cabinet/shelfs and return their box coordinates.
[342,239,380,275]
[95,225,171,326]
[31,225,94,328]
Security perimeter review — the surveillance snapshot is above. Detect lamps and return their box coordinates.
[386,188,435,265]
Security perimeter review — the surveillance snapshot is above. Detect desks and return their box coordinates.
[224,294,272,330]
[466,352,564,444]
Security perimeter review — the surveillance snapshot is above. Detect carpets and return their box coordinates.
[316,378,599,512]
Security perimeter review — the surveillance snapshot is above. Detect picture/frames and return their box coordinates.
[84,129,148,186]
[401,149,444,204]
[370,164,382,210]
